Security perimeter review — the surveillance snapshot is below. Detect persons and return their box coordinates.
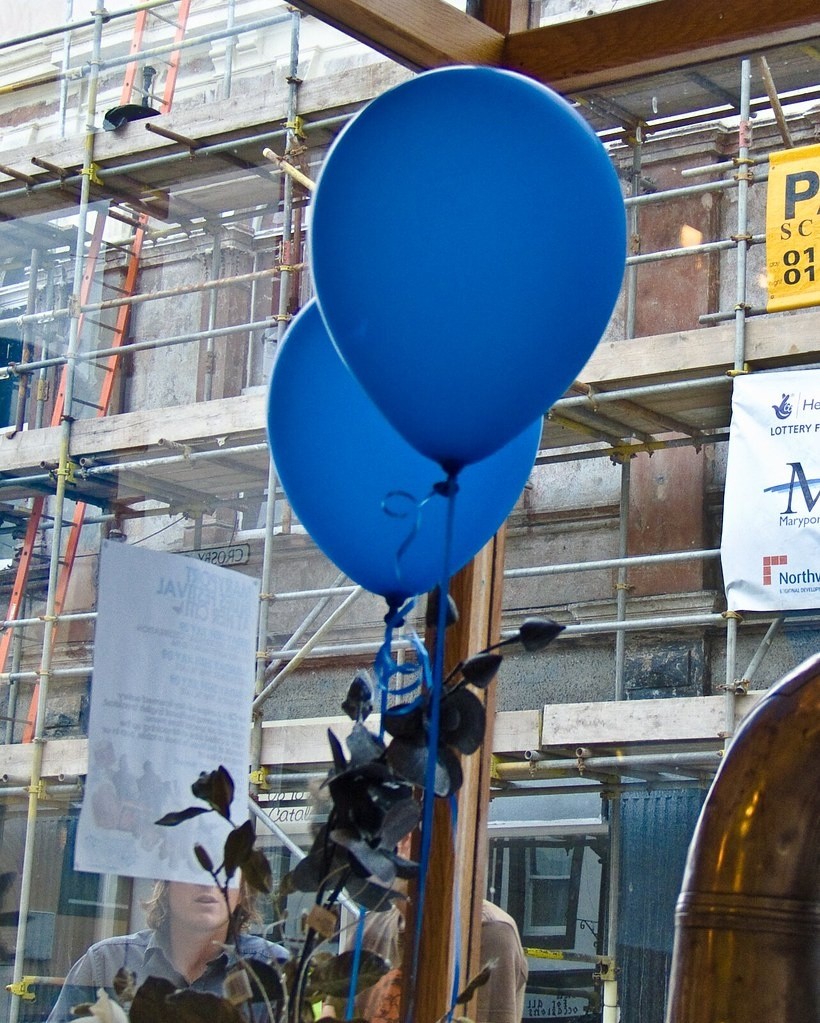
[44,875,293,1023]
[321,831,530,1023]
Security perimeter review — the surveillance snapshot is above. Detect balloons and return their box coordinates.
[307,64,627,497]
[265,293,543,628]
